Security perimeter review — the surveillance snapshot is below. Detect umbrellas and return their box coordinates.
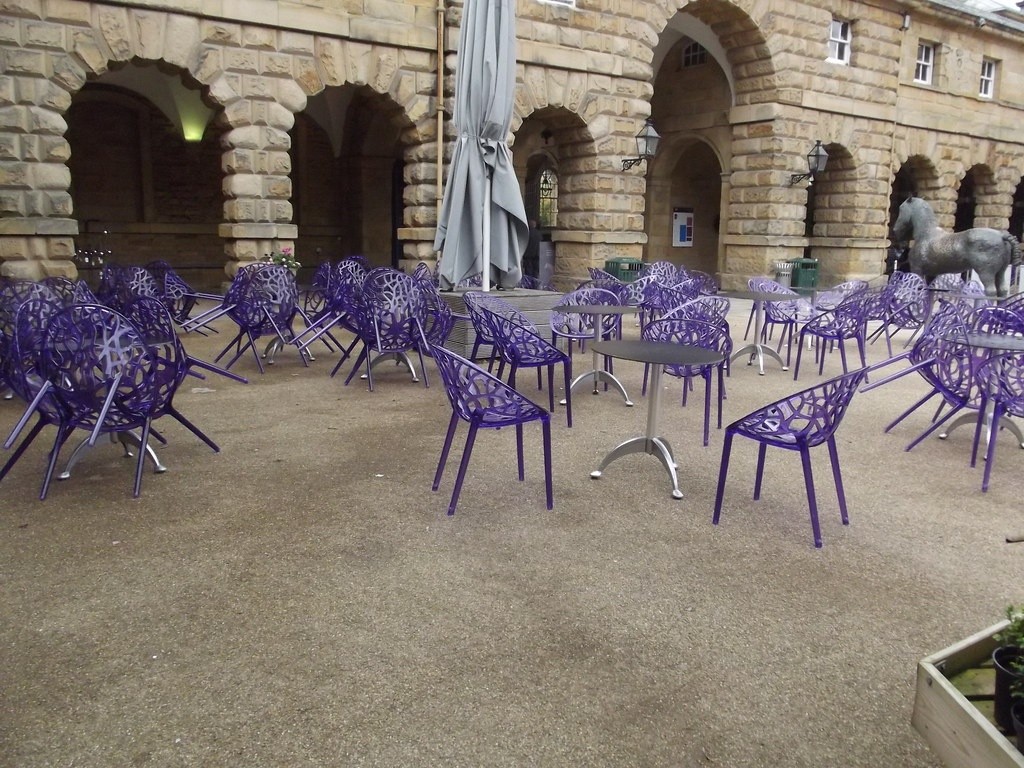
[433,1,530,294]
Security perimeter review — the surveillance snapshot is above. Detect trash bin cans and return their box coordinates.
[607,256,645,306]
[775,261,795,287]
[792,258,819,296]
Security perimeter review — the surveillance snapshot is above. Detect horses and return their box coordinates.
[892,193,1022,333]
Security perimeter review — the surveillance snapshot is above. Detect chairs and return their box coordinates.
[0,251,1024,548]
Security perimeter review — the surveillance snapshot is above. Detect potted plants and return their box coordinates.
[988,602,1024,754]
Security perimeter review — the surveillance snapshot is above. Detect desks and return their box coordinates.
[586,338,725,500]
[712,289,799,375]
[940,333,1024,460]
[947,295,1008,332]
[547,305,643,407]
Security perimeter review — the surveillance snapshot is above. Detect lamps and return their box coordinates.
[973,17,986,27]
[621,117,661,172]
[785,139,828,187]
